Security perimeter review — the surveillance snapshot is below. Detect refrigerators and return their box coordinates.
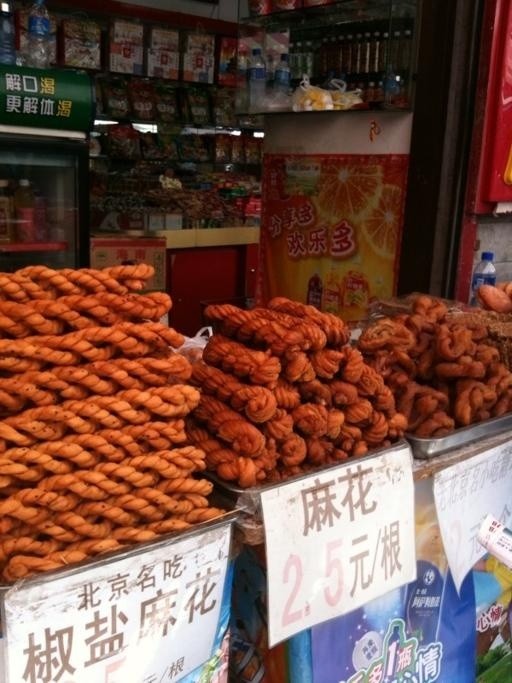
[0,63,96,269]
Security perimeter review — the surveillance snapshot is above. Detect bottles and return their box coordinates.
[274,52,291,101]
[1,174,69,242]
[308,254,378,324]
[0,0,17,63]
[25,0,51,68]
[336,79,406,110]
[248,49,265,107]
[465,251,497,305]
[284,30,409,77]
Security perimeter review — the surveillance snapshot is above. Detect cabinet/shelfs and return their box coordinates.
[234,0,420,116]
